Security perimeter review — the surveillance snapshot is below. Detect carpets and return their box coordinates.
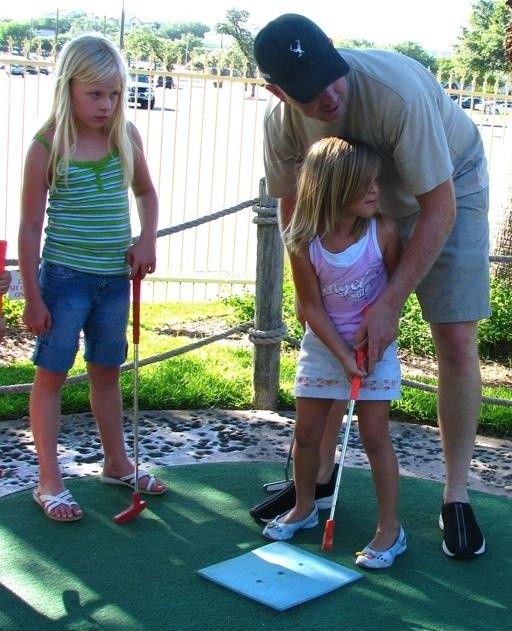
[0,461,512,631]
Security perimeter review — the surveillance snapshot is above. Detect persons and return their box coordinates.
[260,134,409,570]
[16,35,169,524]
[248,11,493,560]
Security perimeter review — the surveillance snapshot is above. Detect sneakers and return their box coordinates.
[250,463,340,524]
[437,500,486,560]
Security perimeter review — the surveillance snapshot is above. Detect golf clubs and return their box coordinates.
[323,304,371,548]
[111,257,145,524]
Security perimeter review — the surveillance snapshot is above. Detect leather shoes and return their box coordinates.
[354,524,409,569]
[262,503,320,542]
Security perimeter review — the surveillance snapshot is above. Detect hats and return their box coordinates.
[253,13,352,105]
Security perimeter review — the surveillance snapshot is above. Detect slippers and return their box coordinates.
[100,468,168,496]
[32,487,84,522]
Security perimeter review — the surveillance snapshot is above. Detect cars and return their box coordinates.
[440,83,511,115]
[6,51,174,111]
